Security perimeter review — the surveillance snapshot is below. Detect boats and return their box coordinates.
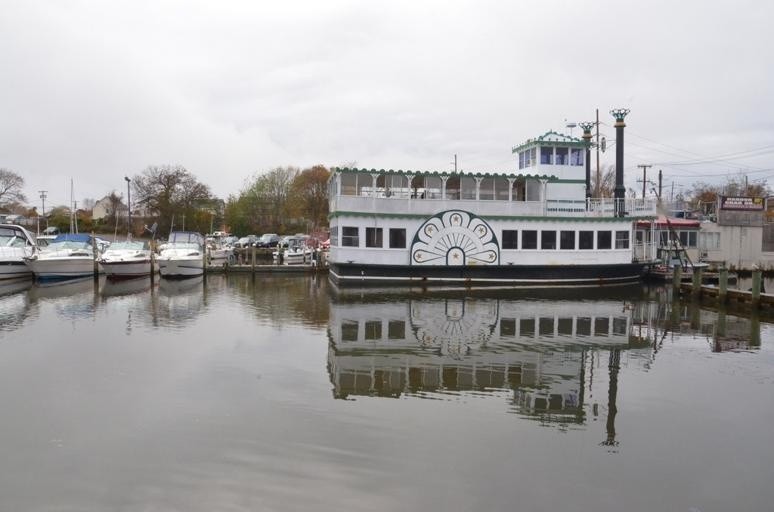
[324,289,654,451]
[0,220,210,278]
[0,274,204,315]
[320,127,669,300]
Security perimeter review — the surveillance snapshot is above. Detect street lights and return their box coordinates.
[123,175,132,233]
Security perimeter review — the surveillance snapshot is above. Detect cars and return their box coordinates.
[203,229,331,265]
